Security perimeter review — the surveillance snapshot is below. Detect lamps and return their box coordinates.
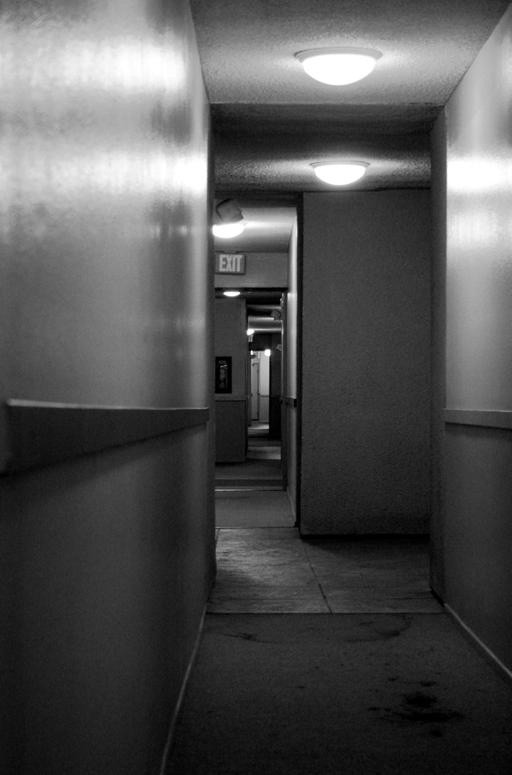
[309,160,370,186]
[293,47,382,86]
[211,197,244,238]
